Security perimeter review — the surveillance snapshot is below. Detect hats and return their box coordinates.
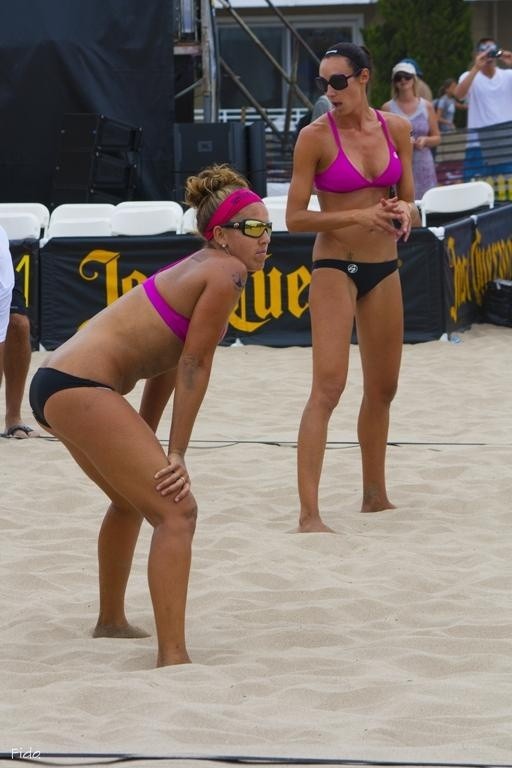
[392,58,423,81]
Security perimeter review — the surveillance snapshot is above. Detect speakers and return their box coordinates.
[47,112,143,217]
[246,121,268,199]
[173,121,247,212]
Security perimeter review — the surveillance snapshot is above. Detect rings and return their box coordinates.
[179,476,186,484]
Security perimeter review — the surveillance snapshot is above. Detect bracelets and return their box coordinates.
[405,199,415,216]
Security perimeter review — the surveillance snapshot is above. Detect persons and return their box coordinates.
[0,224,43,442]
[30,158,275,673]
[284,42,419,534]
[310,38,512,202]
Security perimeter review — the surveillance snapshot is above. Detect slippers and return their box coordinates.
[1,425,34,440]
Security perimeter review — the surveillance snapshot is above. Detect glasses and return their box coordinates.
[478,44,497,51]
[395,75,412,81]
[314,69,362,93]
[221,219,273,238]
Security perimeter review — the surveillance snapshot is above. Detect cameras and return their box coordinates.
[488,49,502,58]
